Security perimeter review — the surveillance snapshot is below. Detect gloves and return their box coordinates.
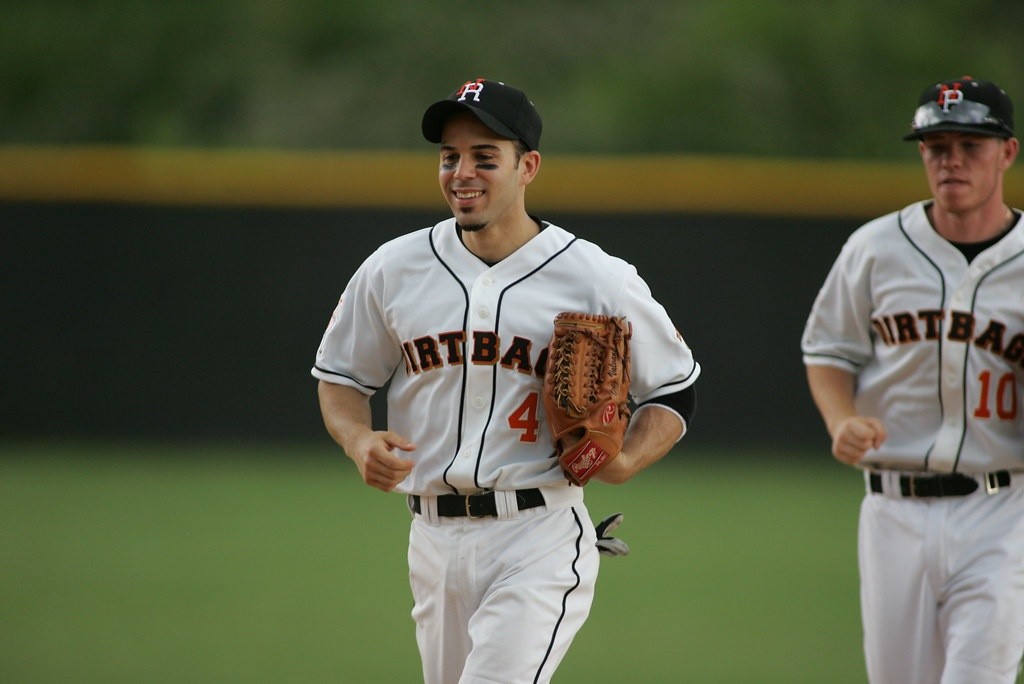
[594,513,630,557]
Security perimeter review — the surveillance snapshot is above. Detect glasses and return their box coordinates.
[912,100,1012,131]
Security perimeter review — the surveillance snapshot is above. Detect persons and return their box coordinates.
[800,75,1024,683]
[311,79,702,684]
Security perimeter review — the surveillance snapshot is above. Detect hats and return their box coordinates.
[421,78,543,150]
[902,75,1016,138]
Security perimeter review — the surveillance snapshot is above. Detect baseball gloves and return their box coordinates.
[540,309,633,486]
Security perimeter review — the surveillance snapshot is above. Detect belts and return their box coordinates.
[411,488,547,519]
[870,469,1010,496]
